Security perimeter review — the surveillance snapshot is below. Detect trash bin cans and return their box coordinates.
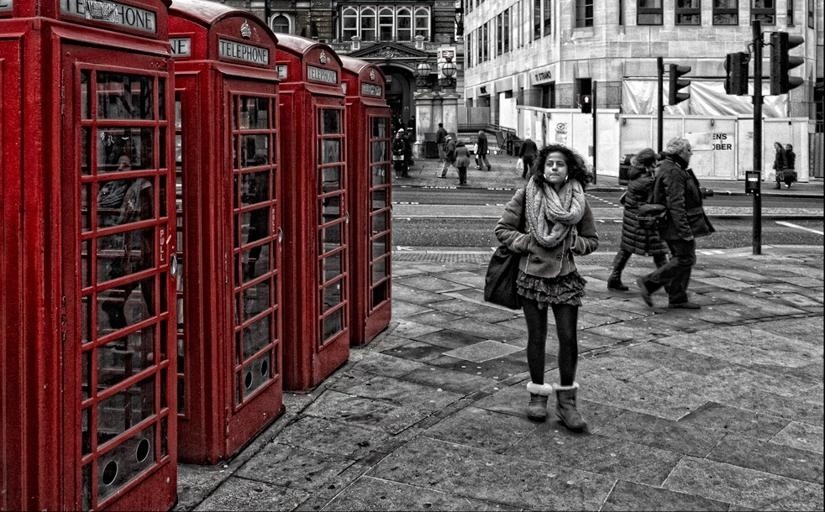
[513,140,524,157]
[423,142,438,159]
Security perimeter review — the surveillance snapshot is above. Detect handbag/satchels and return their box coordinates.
[515,159,524,171]
[483,244,523,310]
[637,204,667,228]
[775,168,797,184]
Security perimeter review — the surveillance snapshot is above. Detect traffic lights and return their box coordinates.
[582,95,592,114]
[769,31,805,96]
[723,51,749,95]
[669,64,690,106]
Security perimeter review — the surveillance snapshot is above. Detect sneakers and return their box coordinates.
[772,184,795,190]
[458,180,467,185]
[437,174,447,178]
[476,165,492,171]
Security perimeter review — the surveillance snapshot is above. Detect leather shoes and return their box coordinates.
[636,278,652,308]
[667,301,700,309]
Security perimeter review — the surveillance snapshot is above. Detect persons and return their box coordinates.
[243,148,270,278]
[773,142,791,189]
[519,137,538,179]
[783,144,795,187]
[494,144,599,430]
[636,137,716,309]
[391,115,491,185]
[606,148,672,294]
[95,151,166,350]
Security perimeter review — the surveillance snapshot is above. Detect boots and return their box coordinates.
[525,380,553,420]
[553,383,588,431]
[395,170,404,179]
[607,266,628,291]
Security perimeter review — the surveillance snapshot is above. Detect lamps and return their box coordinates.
[442,62,457,86]
[417,63,432,85]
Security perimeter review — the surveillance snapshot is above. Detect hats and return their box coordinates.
[637,147,662,162]
[444,135,452,138]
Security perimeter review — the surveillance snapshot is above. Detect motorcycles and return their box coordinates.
[392,124,415,179]
